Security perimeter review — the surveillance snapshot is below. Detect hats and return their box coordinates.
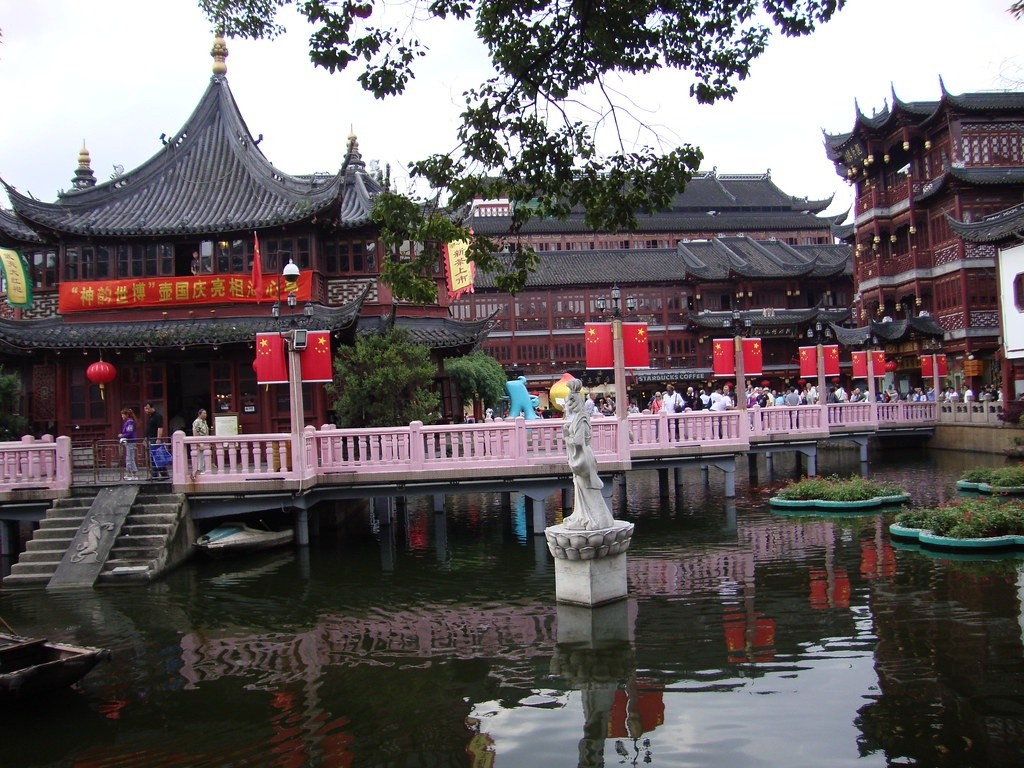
[763,388,769,391]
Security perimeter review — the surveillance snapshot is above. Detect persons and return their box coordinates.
[590,383,1024,443]
[118,408,139,480]
[143,400,170,482]
[191,251,211,275]
[563,379,614,529]
[465,406,552,423]
[192,409,209,475]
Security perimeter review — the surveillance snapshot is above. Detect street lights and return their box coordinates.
[722,307,752,411]
[598,283,635,420]
[858,332,878,402]
[272,249,314,480]
[808,320,831,405]
[925,336,942,401]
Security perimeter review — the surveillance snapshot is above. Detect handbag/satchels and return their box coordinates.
[150,442,172,467]
[674,394,682,413]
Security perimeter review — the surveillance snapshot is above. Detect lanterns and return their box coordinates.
[531,390,539,396]
[726,382,732,387]
[253,358,268,391]
[761,381,769,387]
[87,361,116,400]
[798,379,806,386]
[887,361,896,372]
[832,377,839,384]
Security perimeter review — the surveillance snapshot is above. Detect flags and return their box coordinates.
[609,539,895,739]
[585,322,649,370]
[799,345,840,378]
[256,330,333,384]
[713,338,762,376]
[921,355,948,377]
[852,351,886,378]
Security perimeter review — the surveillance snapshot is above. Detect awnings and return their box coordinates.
[593,383,616,398]
[625,369,711,383]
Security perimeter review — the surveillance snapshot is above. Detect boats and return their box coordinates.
[193,522,296,560]
[0,617,114,706]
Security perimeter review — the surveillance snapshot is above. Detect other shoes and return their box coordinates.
[124,475,133,480]
[145,476,158,481]
[131,476,138,480]
[158,475,170,481]
[200,470,205,474]
